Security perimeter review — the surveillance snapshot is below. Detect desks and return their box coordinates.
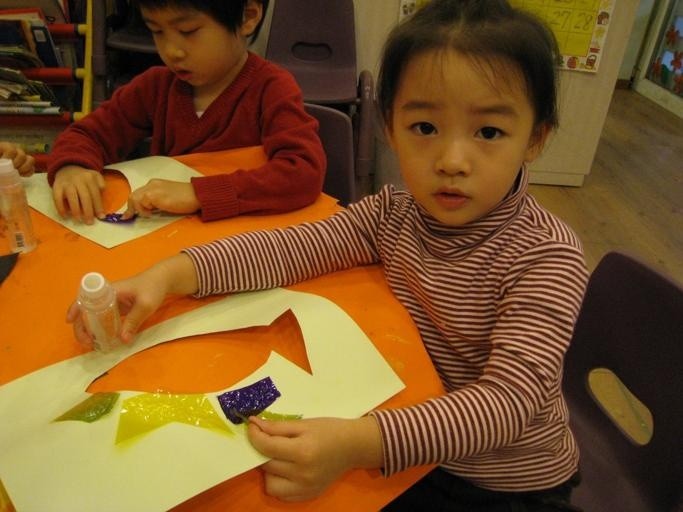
[1,143,463,510]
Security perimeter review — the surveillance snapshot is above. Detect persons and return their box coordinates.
[67,0,588,511]
[0,139,37,177]
[45,0,329,226]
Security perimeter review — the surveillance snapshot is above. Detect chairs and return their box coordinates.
[265,1,375,181]
[563,249,683,511]
[295,103,356,206]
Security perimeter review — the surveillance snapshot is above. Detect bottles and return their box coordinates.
[75,271,124,354]
[0,158,38,255]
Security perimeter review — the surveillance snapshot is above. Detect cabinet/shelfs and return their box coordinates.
[100,1,163,99]
[352,0,642,187]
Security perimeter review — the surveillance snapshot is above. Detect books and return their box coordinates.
[1,0,80,117]
